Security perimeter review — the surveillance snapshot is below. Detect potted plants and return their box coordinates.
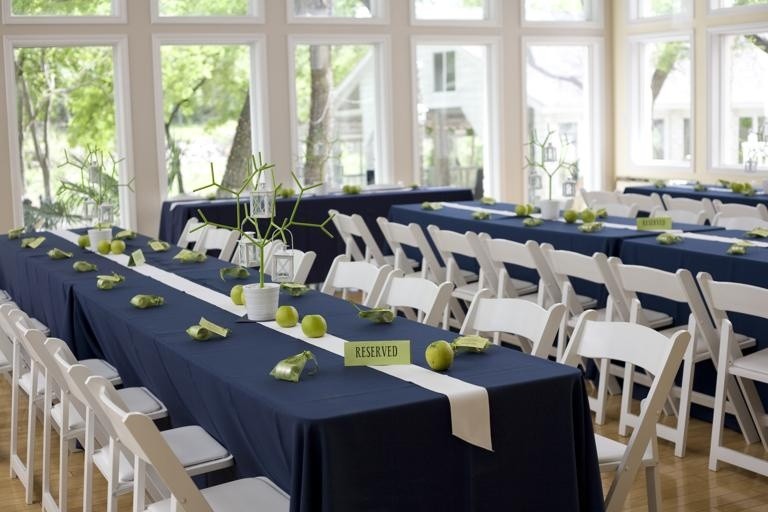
[55,141,138,253]
[520,122,579,221]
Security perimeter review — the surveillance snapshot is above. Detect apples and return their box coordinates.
[282,188,295,197]
[515,204,533,215]
[581,210,594,223]
[99,240,125,254]
[731,181,754,195]
[230,285,242,305]
[78,236,90,249]
[565,210,577,222]
[302,314,327,338]
[426,340,454,370]
[206,193,216,201]
[342,184,361,193]
[276,306,299,328]
